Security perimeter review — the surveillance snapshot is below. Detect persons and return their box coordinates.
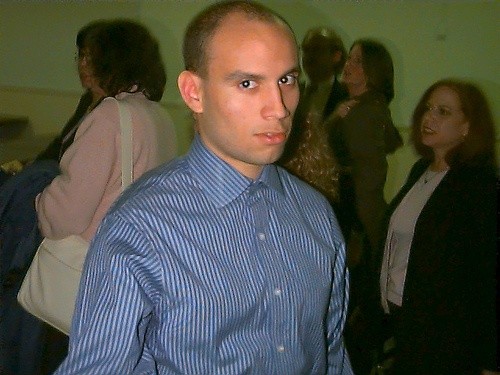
[273,24,402,247]
[377,79,500,375]
[0,18,179,334]
[52,0,351,375]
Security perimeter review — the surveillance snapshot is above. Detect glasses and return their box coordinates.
[74,52,91,63]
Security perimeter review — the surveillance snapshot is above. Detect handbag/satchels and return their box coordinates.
[17,234,90,337]
